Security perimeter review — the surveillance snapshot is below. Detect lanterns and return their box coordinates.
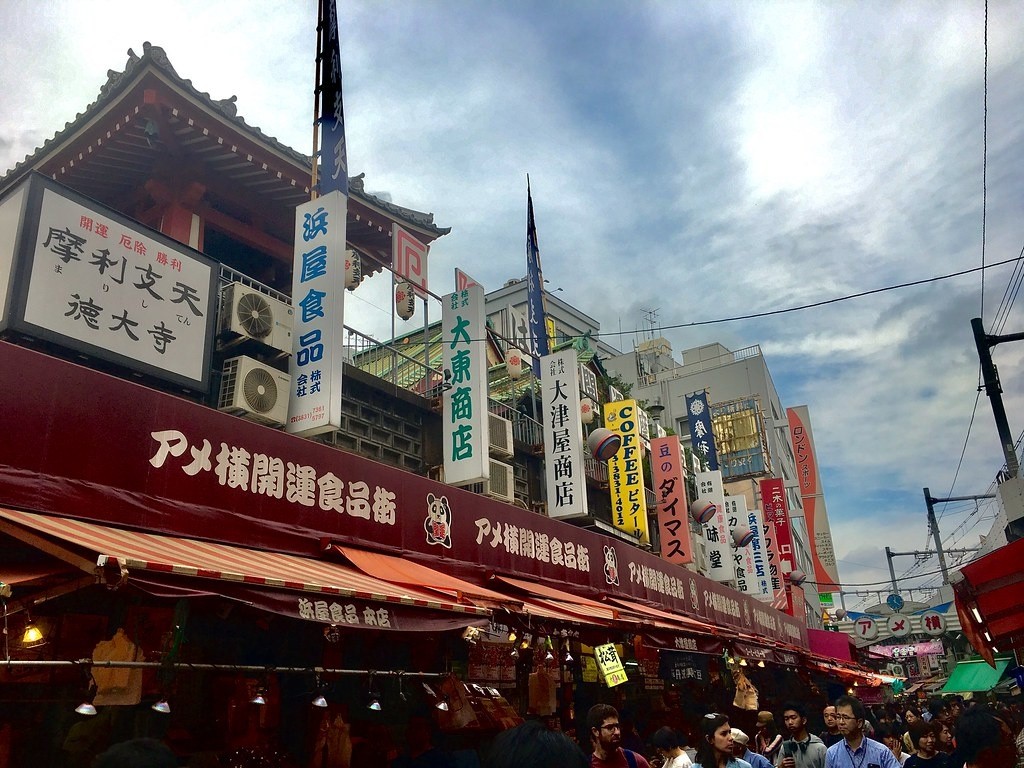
[580,398,593,424]
[506,348,521,381]
[338,250,361,291]
[396,283,414,320]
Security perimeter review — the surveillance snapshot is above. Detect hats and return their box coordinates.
[756,711,775,727]
[729,728,750,747]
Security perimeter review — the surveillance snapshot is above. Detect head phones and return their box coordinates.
[790,732,810,752]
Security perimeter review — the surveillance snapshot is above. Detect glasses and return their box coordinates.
[835,714,856,721]
[704,712,722,720]
[600,723,620,730]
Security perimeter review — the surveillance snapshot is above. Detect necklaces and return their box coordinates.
[844,738,867,768]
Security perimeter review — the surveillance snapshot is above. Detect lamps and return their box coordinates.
[789,570,807,586]
[545,635,554,659]
[836,609,846,619]
[152,659,172,713]
[250,670,270,705]
[21,608,47,649]
[75,665,100,716]
[419,676,450,711]
[691,499,716,524]
[587,427,621,462]
[507,627,517,641]
[727,653,734,664]
[518,632,529,649]
[758,655,765,667]
[311,673,328,707]
[732,526,755,547]
[367,669,382,710]
[739,658,747,666]
[564,637,574,661]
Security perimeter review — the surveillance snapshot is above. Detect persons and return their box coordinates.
[92,737,176,768]
[650,713,775,768]
[587,704,651,768]
[821,706,844,748]
[484,721,589,768]
[389,711,456,768]
[864,694,1024,768]
[823,695,902,768]
[755,711,784,766]
[777,703,827,768]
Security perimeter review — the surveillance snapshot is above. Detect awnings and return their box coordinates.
[0,506,490,624]
[332,545,524,612]
[942,658,1013,693]
[905,683,924,693]
[497,575,881,686]
